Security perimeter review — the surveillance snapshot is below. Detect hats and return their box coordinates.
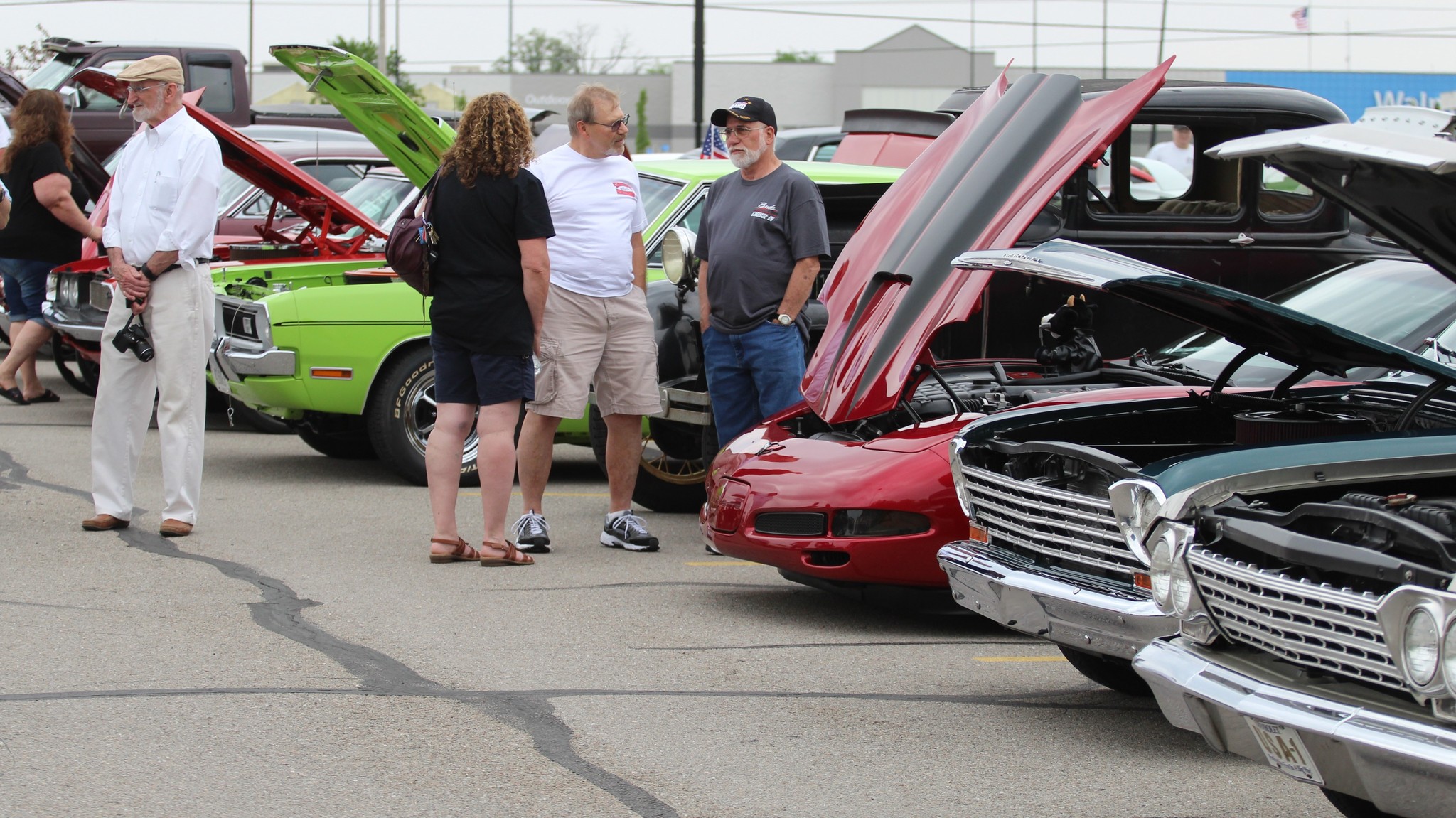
[115,55,185,84]
[711,97,777,137]
[1174,125,1189,130]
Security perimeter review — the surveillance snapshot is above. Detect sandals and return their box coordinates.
[428,535,481,561]
[481,538,533,567]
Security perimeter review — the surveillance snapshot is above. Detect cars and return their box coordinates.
[195,42,904,484]
[0,40,1414,362]
[1107,127,1455,818]
[940,227,1453,699]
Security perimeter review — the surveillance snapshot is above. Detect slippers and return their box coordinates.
[23,388,60,403]
[0,386,25,405]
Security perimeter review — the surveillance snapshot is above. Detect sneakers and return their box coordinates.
[510,509,551,550]
[599,510,659,551]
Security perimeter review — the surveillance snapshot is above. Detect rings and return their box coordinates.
[122,291,126,294]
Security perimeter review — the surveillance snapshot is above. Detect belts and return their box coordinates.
[131,257,208,275]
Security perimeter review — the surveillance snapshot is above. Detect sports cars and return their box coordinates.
[703,51,1455,599]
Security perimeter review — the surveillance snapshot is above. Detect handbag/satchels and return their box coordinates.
[672,317,706,371]
[385,161,452,297]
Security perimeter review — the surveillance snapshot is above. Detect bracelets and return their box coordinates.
[87,224,94,237]
[141,262,158,281]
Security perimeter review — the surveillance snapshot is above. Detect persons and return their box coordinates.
[0,114,15,172]
[1145,125,1195,183]
[409,93,552,565]
[693,96,830,556]
[80,55,224,538]
[0,89,104,406]
[0,179,12,229]
[515,86,660,553]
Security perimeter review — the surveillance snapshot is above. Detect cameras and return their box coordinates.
[111,323,154,363]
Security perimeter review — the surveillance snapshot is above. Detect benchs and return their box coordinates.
[1159,198,1237,217]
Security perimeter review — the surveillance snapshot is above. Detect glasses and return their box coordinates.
[718,127,768,141]
[126,83,179,93]
[582,114,629,132]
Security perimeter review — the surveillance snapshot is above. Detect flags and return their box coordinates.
[701,122,731,159]
[1292,5,1312,31]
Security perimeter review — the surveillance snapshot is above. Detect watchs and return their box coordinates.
[0,189,6,202]
[774,313,793,326]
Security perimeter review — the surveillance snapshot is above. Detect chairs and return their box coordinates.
[328,177,362,197]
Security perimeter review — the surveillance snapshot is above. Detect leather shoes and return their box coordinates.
[83,512,131,529]
[159,519,193,536]
